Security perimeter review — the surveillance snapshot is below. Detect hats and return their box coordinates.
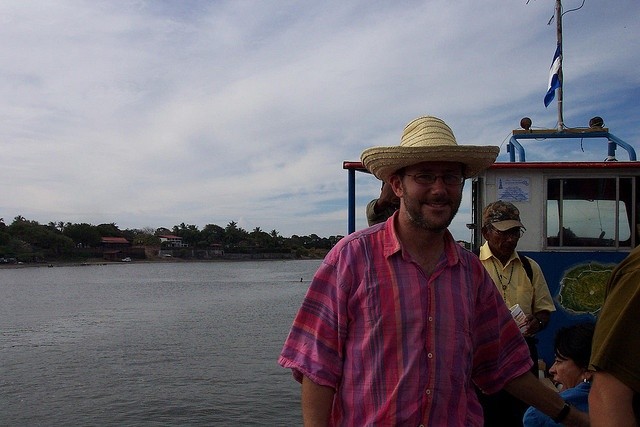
[361,115,501,184]
[482,200,526,232]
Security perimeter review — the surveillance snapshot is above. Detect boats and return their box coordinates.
[343,0,640,427]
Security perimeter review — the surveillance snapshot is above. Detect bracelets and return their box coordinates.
[553,401,570,424]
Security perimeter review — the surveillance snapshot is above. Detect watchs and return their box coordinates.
[535,317,544,328]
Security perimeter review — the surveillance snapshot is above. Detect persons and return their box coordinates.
[366,179,400,227]
[277,117,589,426]
[588,248,640,427]
[472,201,556,426]
[523,322,596,426]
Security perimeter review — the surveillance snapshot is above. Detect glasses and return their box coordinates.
[397,168,465,187]
[490,228,525,238]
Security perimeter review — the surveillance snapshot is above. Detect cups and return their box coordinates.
[509,303,535,340]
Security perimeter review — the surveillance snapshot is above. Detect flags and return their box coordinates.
[544,47,561,108]
